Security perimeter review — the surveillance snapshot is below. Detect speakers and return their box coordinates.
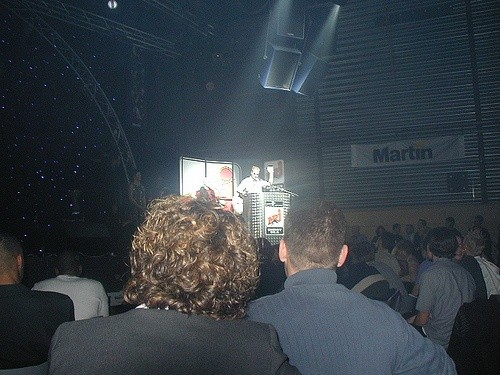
[257,0,340,96]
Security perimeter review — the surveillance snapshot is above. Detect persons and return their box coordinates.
[127,170,148,211]
[248,238,288,298]
[29,247,109,319]
[241,191,457,375]
[0,233,75,370]
[47,193,304,375]
[336,214,500,375]
